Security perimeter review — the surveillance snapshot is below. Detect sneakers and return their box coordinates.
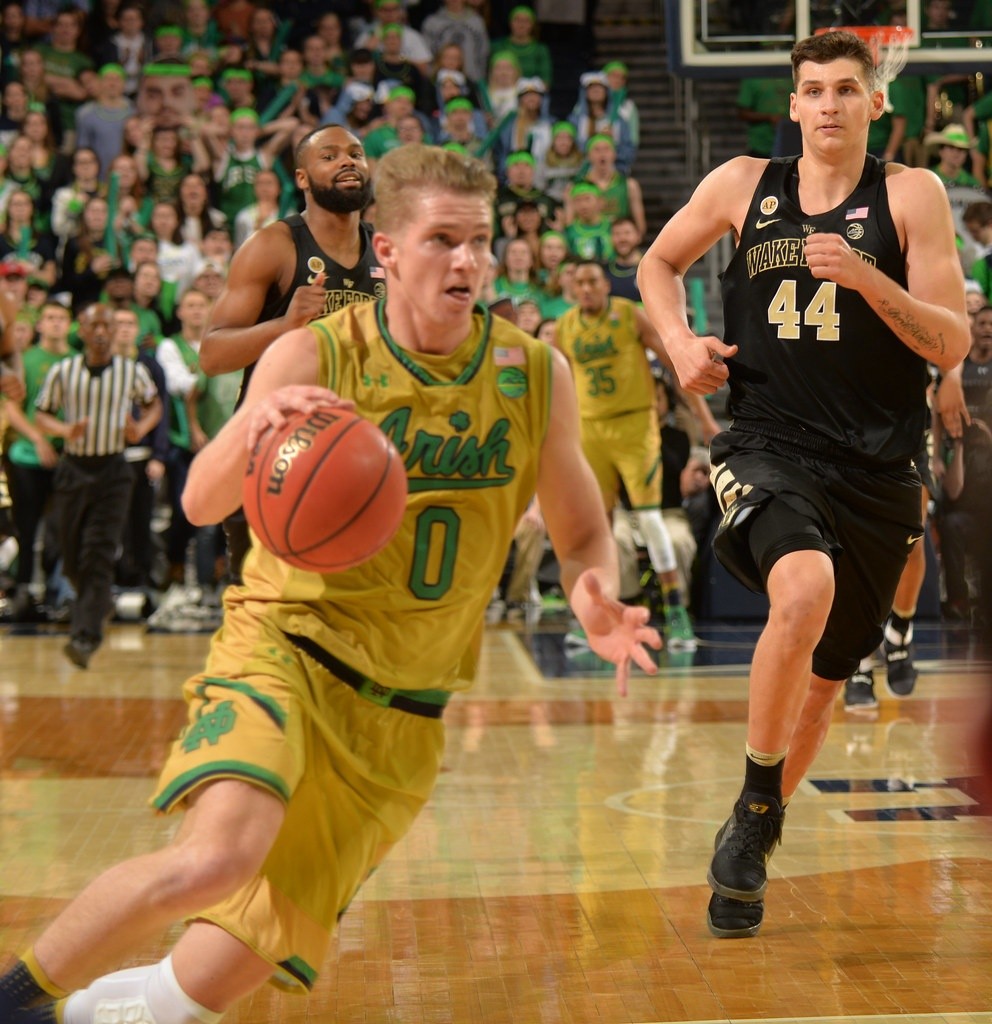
[708,792,785,903]
[881,616,918,697]
[564,629,587,644]
[146,581,224,631]
[707,815,766,936]
[843,657,875,710]
[663,604,698,654]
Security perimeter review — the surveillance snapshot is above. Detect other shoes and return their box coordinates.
[63,639,90,669]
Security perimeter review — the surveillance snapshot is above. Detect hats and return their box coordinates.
[922,123,980,149]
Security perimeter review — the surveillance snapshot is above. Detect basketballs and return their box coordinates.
[239,404,408,574]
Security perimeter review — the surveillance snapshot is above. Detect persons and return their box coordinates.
[547,259,724,651]
[1,144,662,1024]
[740,1,992,712]
[34,302,161,670]
[1,0,713,632]
[637,31,973,939]
